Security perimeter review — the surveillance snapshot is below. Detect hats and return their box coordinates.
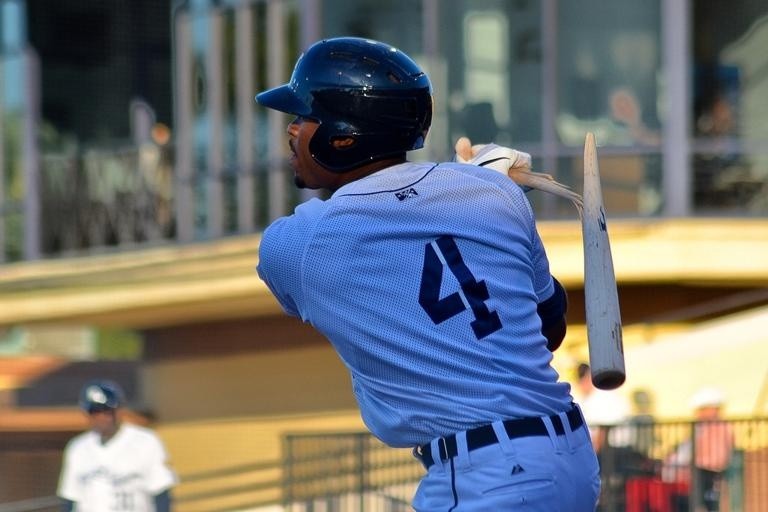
[80,379,124,413]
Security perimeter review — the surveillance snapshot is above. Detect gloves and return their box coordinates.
[454,136,532,177]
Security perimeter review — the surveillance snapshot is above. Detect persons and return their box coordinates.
[662,388,737,512]
[569,366,627,512]
[620,389,673,512]
[252,33,605,511]
[54,377,177,512]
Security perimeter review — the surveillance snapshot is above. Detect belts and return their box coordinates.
[421,402,584,471]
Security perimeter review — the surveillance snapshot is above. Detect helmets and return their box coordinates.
[253,36,435,175]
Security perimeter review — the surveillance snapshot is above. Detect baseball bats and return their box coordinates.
[454,133,625,389]
